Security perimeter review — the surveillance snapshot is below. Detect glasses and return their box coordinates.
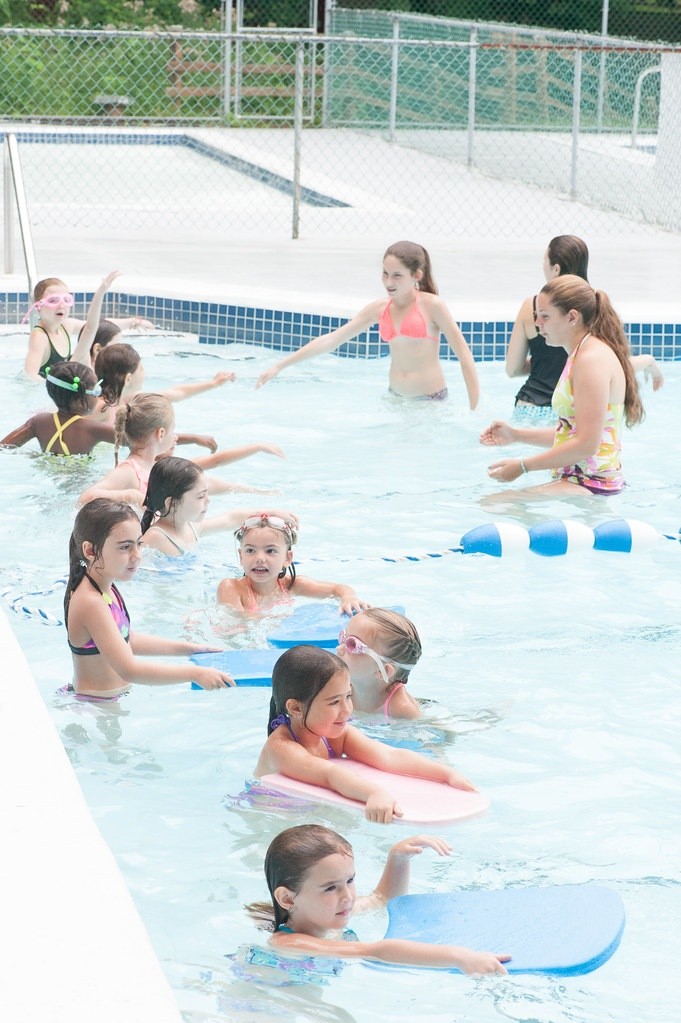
[337,630,390,685]
[39,293,75,307]
[240,513,292,548]
[93,379,104,396]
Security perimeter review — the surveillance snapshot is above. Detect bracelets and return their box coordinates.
[519,459,528,474]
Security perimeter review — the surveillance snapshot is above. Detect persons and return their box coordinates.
[506,234,663,426]
[63,496,235,702]
[477,273,645,507]
[0,268,372,641]
[230,825,511,989]
[334,606,424,722]
[255,239,480,412]
[235,646,477,826]
